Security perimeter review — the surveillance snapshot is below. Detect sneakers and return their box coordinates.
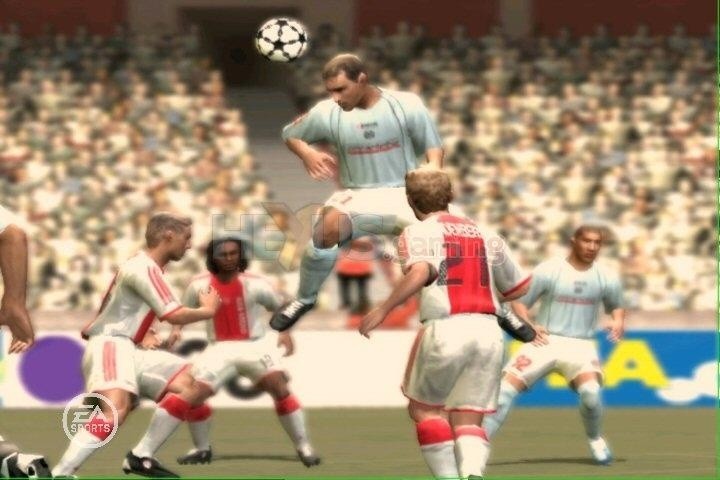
[271,298,316,331]
[589,437,612,463]
[122,445,320,478]
[497,312,535,343]
